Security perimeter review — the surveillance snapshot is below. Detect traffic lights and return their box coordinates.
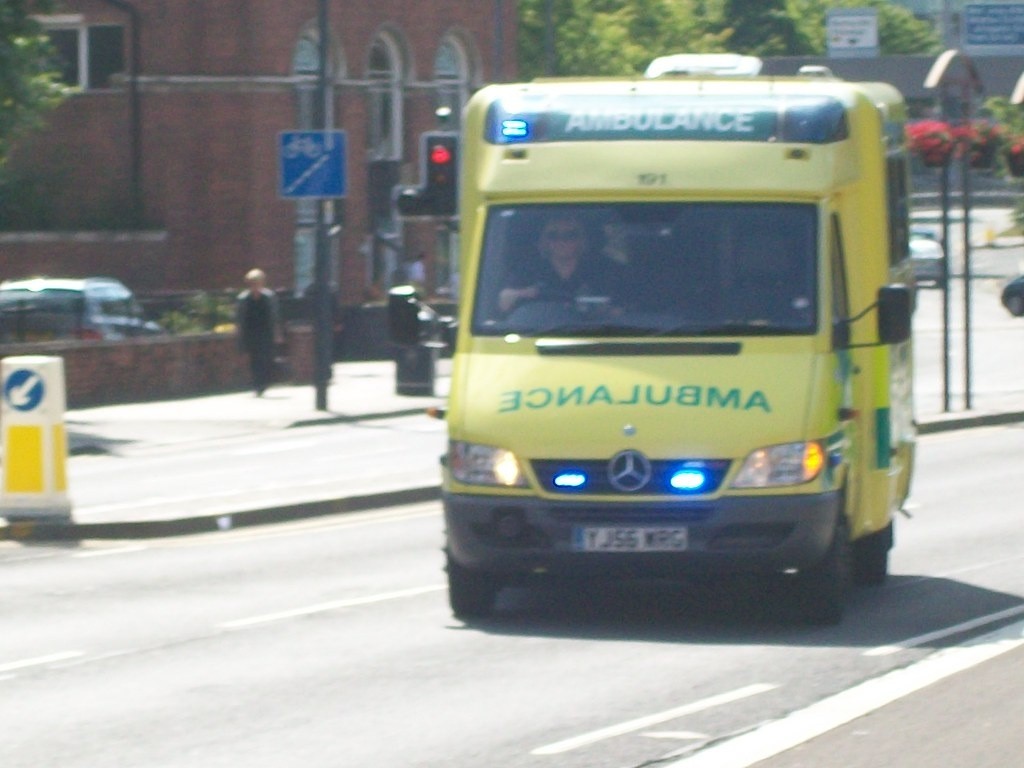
[33,17,91,95]
[420,131,461,215]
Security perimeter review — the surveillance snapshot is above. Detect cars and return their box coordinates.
[1,275,168,341]
[908,229,945,283]
[1000,276,1024,318]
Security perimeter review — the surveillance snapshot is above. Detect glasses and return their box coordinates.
[540,229,587,241]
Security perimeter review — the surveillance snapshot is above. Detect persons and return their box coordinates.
[301,268,344,384]
[495,216,637,320]
[233,269,281,399]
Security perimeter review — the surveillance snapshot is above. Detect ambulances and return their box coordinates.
[436,53,921,625]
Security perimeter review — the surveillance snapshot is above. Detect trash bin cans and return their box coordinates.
[393,342,446,397]
